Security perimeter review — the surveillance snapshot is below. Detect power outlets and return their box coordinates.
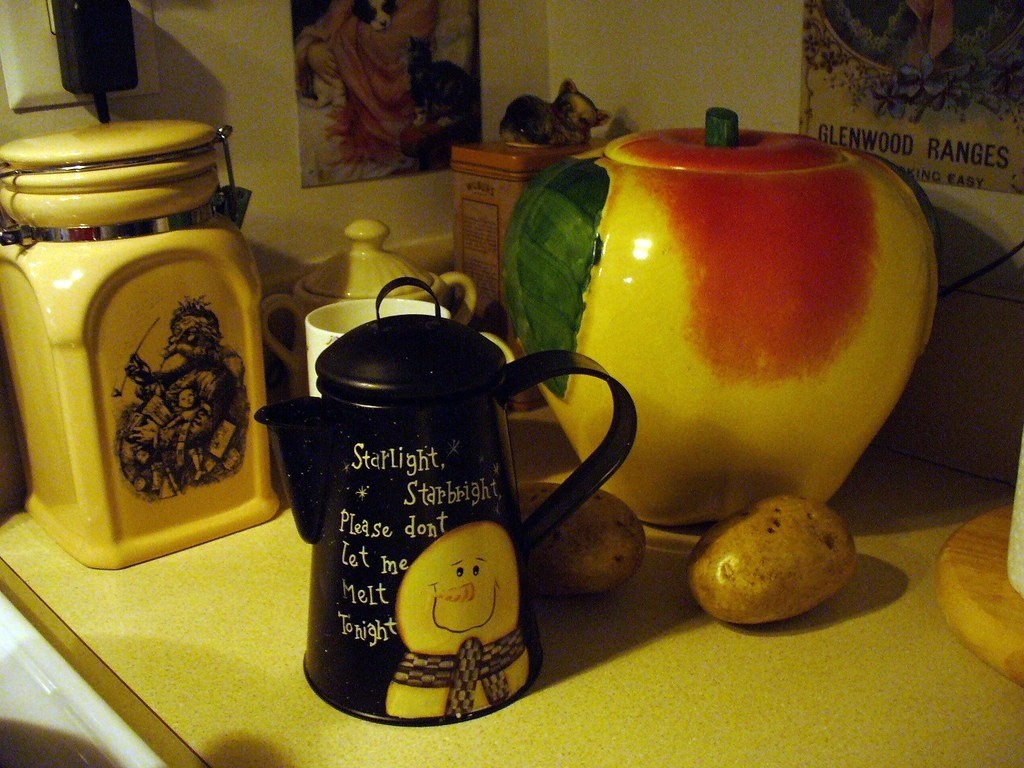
[0,0,161,111]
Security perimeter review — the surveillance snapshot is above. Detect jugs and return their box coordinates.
[254,274,638,727]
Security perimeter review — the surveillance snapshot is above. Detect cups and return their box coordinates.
[306,298,514,398]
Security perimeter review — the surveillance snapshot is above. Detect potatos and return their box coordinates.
[515,483,645,594]
[687,493,857,623]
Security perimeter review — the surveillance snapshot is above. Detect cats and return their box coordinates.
[499,77,612,148]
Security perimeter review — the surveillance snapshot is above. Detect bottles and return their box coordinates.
[1,119,279,571]
[259,215,477,399]
